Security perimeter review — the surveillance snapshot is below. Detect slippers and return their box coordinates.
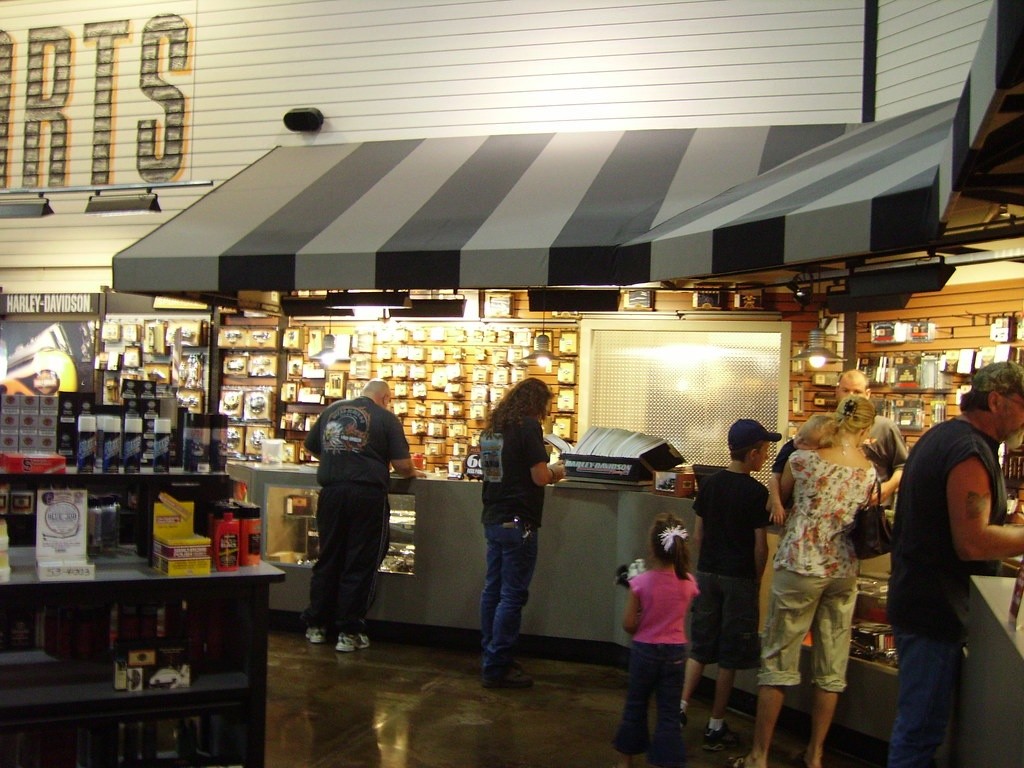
[724,755,748,768]
[790,750,808,768]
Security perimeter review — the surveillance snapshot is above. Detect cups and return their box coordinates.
[261,440,286,469]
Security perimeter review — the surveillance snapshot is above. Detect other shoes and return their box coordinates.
[482,667,534,688]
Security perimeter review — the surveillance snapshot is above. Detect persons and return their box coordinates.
[303,378,427,652]
[838,370,909,506]
[479,378,565,688]
[613,515,698,768]
[729,396,875,768]
[887,362,1024,768]
[769,414,837,527]
[678,420,782,750]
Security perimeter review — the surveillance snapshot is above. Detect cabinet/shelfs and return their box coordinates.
[0,548,289,768]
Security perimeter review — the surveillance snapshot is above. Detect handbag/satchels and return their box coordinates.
[851,481,893,560]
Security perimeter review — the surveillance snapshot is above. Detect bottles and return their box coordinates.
[283,488,320,560]
[73,412,228,475]
[87,495,121,559]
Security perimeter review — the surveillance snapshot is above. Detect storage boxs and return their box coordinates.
[0,450,68,474]
[150,498,211,575]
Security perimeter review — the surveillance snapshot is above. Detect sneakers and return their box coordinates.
[305,626,327,643]
[678,709,688,728]
[702,720,739,751]
[335,632,369,652]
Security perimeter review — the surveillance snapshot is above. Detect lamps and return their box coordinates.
[0,192,55,219]
[309,290,350,366]
[787,262,848,367]
[786,267,814,306]
[518,287,566,366]
[84,187,162,212]
[843,245,957,299]
[406,292,467,318]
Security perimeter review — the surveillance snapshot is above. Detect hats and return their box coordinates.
[728,419,782,450]
[972,362,1024,397]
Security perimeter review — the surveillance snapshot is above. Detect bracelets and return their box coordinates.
[549,468,554,484]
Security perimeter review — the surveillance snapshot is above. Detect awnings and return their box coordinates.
[111,0,1024,308]
